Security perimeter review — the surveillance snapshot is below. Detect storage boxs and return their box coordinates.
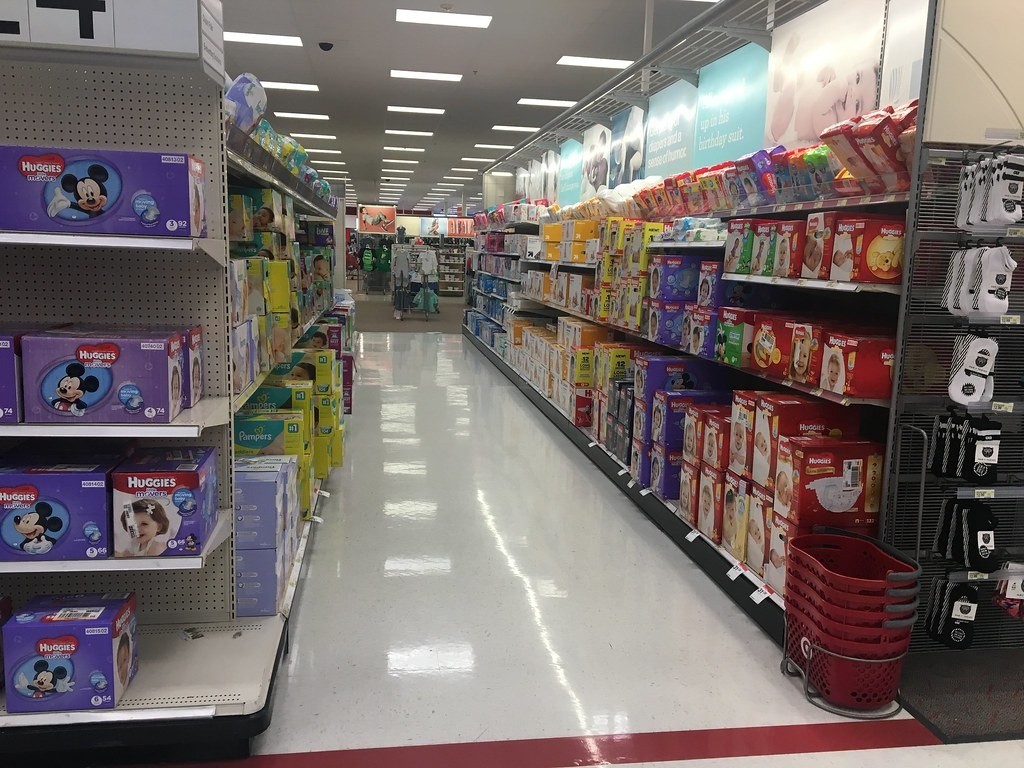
[463,98,919,599]
[1,96,354,712]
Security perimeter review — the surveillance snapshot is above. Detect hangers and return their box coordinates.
[366,235,430,293]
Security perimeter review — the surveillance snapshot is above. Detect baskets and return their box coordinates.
[782,526,923,711]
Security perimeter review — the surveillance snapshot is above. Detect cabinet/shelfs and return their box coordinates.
[0,46,290,768]
[396,235,474,292]
[460,190,911,657]
[225,120,338,620]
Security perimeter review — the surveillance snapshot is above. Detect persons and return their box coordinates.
[630,366,662,489]
[723,490,738,547]
[683,424,696,462]
[191,176,204,236]
[116,631,131,690]
[777,471,793,508]
[680,476,690,514]
[252,188,332,394]
[705,431,717,466]
[361,206,394,235]
[646,268,712,355]
[803,236,823,269]
[753,239,765,270]
[770,546,786,579]
[169,358,205,416]
[699,487,714,533]
[118,501,173,559]
[835,249,855,269]
[766,64,878,146]
[774,237,789,277]
[724,237,741,272]
[749,517,765,552]
[790,333,811,382]
[754,432,771,462]
[731,421,745,467]
[821,354,844,394]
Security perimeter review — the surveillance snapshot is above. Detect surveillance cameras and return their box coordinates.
[319,43,334,51]
[651,65,661,73]
[386,179,390,182]
